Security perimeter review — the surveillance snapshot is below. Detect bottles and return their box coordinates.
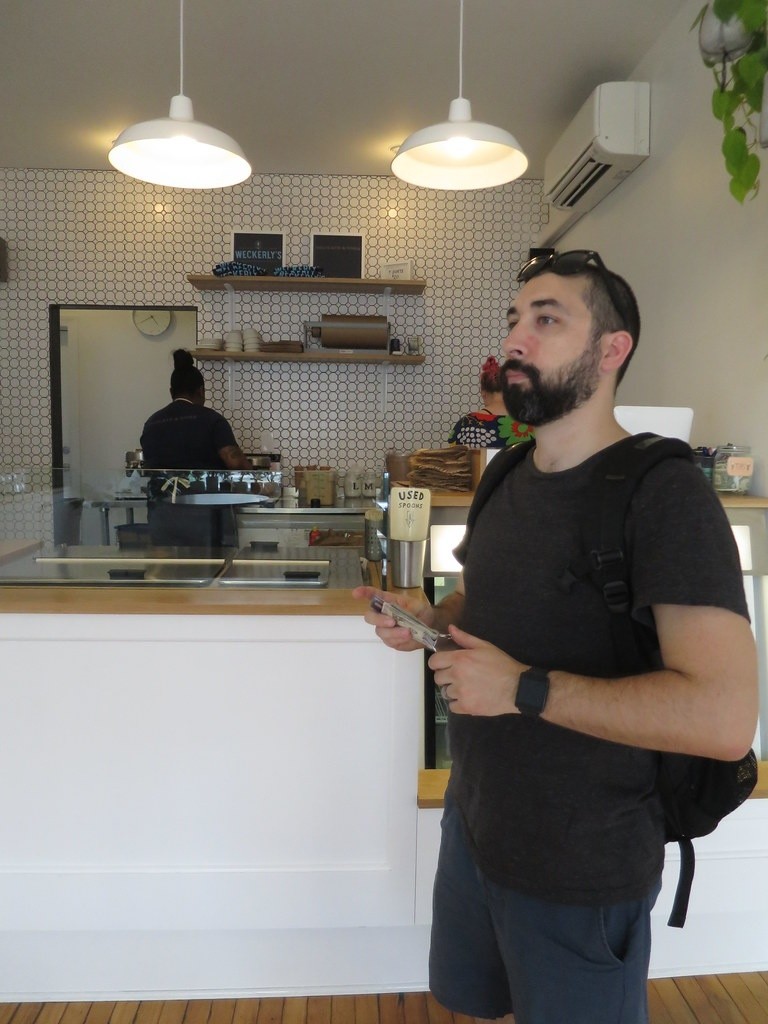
[294,466,306,500]
[318,467,333,506]
[344,471,362,498]
[713,446,754,494]
[306,465,318,505]
[362,472,376,498]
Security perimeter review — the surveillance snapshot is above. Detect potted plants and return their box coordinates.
[689,0,768,202]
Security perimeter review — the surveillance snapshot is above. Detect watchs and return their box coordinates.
[514,664,554,719]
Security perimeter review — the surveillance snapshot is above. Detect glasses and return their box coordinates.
[517,250,609,283]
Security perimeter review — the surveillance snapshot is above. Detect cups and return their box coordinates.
[693,451,714,482]
[390,339,400,353]
[386,538,428,588]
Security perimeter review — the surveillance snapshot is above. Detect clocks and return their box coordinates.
[132,310,171,336]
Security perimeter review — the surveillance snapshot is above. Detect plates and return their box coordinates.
[194,344,220,349]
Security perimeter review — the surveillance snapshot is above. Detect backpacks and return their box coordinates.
[467,434,761,928]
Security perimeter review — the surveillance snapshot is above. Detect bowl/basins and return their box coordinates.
[225,328,262,352]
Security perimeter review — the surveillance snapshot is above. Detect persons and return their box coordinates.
[446,356,535,450]
[139,349,254,548]
[351,258,759,1023]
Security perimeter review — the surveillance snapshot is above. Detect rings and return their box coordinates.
[442,684,455,701]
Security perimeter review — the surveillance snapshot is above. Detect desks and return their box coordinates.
[91,500,149,546]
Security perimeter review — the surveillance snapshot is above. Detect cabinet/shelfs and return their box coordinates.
[235,515,366,547]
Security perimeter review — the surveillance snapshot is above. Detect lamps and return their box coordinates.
[108,0,252,189]
[389,0,528,190]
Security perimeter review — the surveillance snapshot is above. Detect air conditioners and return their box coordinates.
[544,80,651,214]
